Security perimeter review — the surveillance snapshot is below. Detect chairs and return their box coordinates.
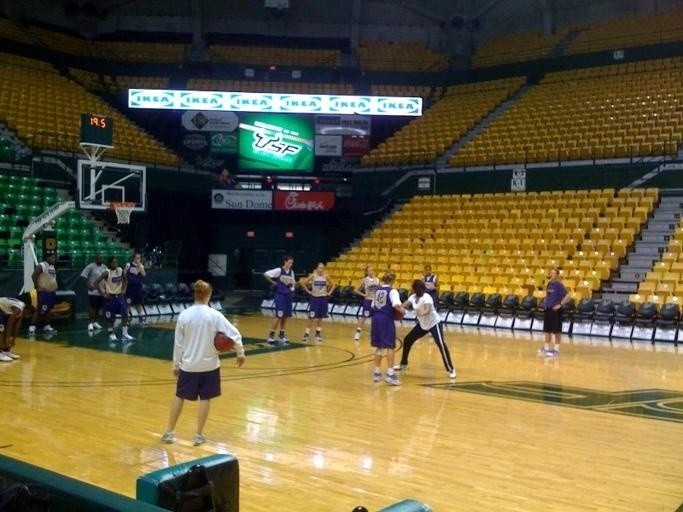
[291,188,682,328]
[448,10,681,58]
[1,41,179,163]
[0,176,151,267]
[179,38,446,100]
[359,58,681,164]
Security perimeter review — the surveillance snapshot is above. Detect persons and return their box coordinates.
[537,268,571,356]
[353,262,455,385]
[263,254,296,342]
[302,262,336,341]
[160,279,246,446]
[0,250,148,362]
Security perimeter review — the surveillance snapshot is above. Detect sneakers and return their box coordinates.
[539,348,559,357]
[373,365,406,385]
[161,433,173,442]
[194,435,206,445]
[449,368,455,378]
[0,351,20,361]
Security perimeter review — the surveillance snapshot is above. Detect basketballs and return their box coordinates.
[214,333,234,352]
[394,308,404,320]
